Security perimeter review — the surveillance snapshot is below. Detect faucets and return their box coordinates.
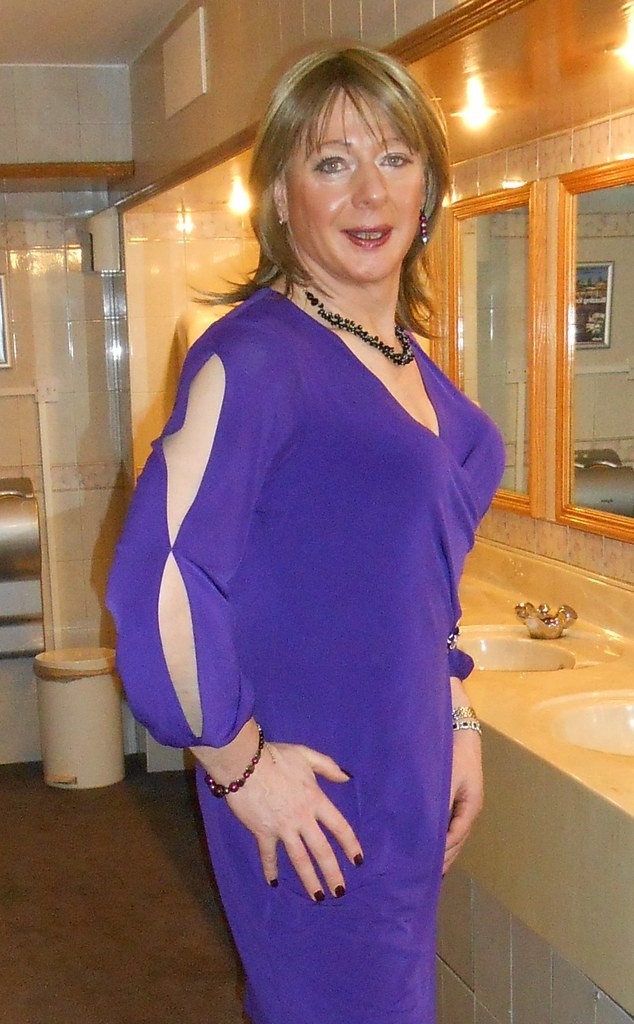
[514,602,541,639]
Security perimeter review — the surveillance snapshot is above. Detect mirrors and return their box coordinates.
[554,158,634,546]
[438,179,549,521]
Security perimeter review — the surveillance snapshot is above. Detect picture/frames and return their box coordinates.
[572,259,613,351]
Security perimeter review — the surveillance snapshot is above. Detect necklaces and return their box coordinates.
[303,288,416,366]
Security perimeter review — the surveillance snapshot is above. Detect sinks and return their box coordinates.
[452,617,623,673]
[531,683,634,756]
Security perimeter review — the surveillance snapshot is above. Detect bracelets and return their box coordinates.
[451,707,478,721]
[453,719,483,736]
[204,722,264,799]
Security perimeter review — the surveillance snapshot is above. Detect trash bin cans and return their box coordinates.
[33,644,129,791]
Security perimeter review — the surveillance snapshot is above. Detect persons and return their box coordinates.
[104,41,507,1023]
